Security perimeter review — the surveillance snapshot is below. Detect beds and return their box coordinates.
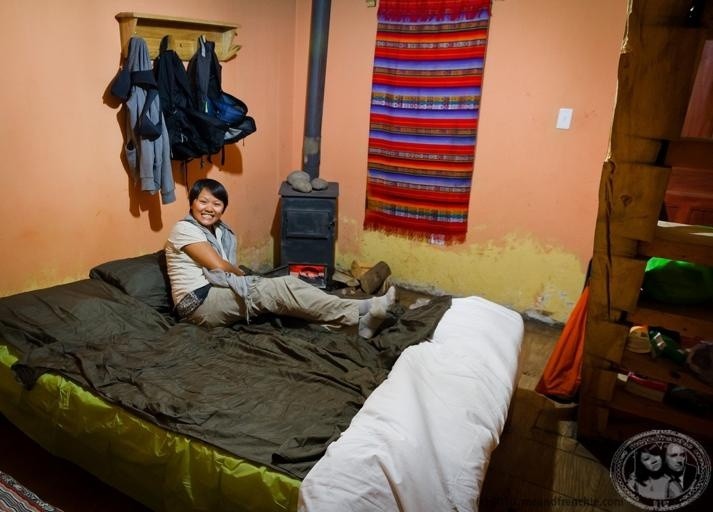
[1,264,525,512]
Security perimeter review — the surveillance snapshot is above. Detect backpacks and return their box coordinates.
[110,35,257,160]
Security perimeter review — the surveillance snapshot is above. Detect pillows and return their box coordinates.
[92,250,167,303]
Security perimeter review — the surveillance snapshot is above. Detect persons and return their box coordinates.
[630,443,682,498]
[664,443,696,491]
[165,179,396,339]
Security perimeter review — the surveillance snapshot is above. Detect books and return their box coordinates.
[625,372,667,402]
